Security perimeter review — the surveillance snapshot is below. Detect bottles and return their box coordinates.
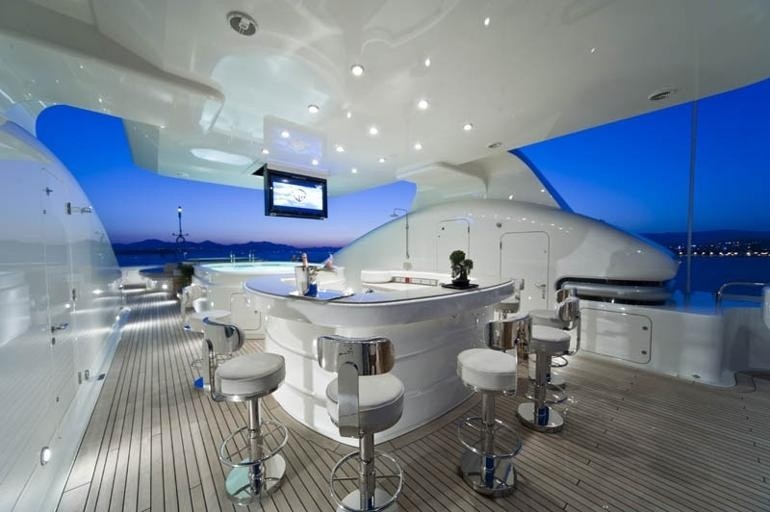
[301,253,308,265]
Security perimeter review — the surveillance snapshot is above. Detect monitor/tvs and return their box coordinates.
[263,164,328,221]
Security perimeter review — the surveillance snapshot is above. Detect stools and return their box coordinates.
[491,279,525,320]
[199,318,290,478]
[514,296,581,434]
[526,287,577,391]
[315,331,406,512]
[455,314,534,496]
[180,286,231,389]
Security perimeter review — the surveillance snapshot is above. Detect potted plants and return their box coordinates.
[450,250,473,287]
